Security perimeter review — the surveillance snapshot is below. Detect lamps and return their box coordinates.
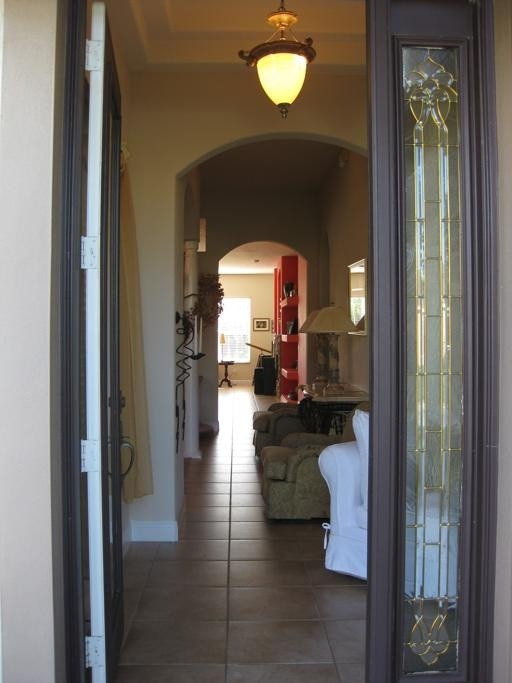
[299,308,360,395]
[231,1,323,126]
[219,333,226,362]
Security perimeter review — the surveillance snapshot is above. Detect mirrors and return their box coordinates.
[348,258,369,336]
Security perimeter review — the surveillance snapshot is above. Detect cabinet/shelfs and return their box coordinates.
[275,253,299,403]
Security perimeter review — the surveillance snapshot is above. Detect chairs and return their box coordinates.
[252,399,370,581]
[319,405,462,603]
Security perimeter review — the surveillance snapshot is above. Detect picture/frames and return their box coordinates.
[252,318,270,332]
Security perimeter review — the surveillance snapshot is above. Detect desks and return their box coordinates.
[218,362,235,388]
[300,383,370,434]
